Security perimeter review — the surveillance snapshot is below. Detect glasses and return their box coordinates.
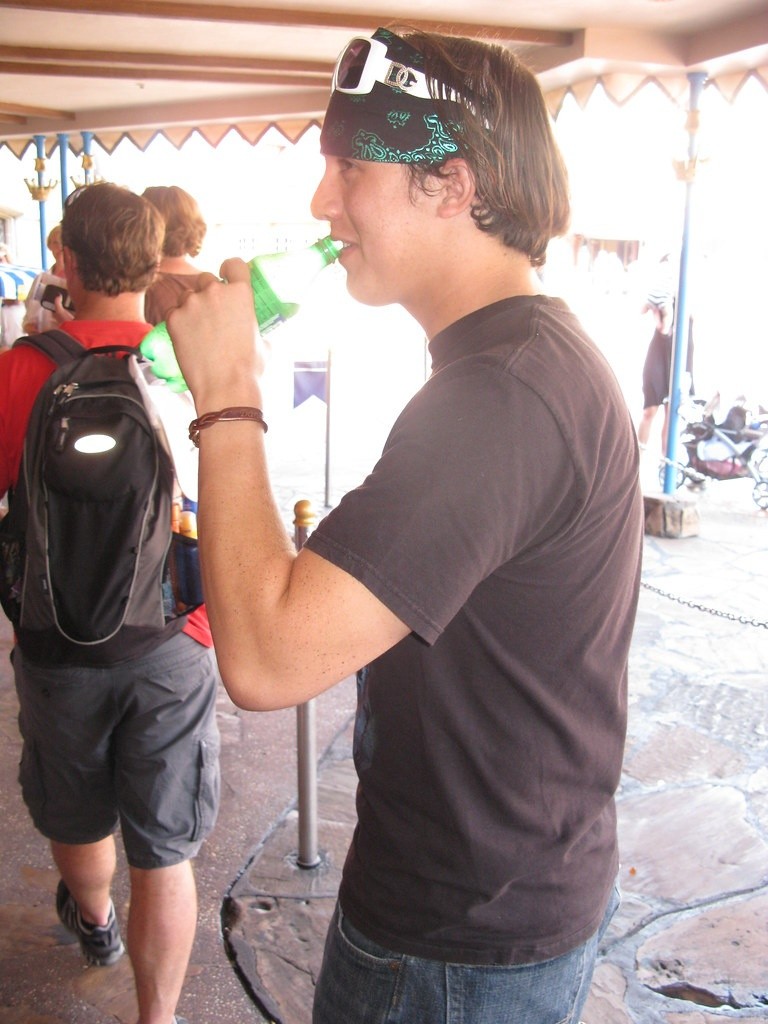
[330,36,494,131]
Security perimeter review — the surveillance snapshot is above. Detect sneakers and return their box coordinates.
[56,879,126,965]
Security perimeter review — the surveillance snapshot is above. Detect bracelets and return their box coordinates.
[189,407,268,448]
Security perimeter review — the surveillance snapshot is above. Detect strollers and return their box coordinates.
[657,396,768,510]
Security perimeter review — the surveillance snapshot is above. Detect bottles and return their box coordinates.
[137,237,349,391]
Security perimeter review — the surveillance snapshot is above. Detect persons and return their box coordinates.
[639,252,694,457]
[166,29,643,1024]
[0,180,219,1024]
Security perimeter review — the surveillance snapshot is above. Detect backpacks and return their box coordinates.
[0,329,206,668]
